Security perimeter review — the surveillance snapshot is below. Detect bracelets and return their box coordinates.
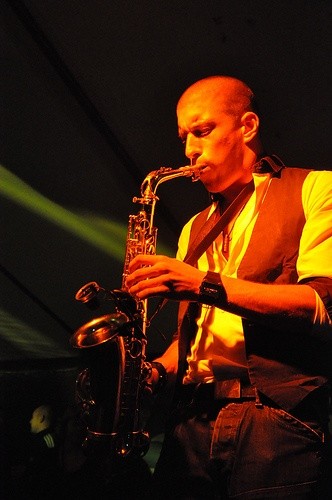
[198,273,224,306]
[149,361,167,389]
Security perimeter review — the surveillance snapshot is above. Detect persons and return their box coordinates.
[125,76,332,500]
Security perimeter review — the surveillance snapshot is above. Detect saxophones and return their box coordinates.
[69,163,205,469]
